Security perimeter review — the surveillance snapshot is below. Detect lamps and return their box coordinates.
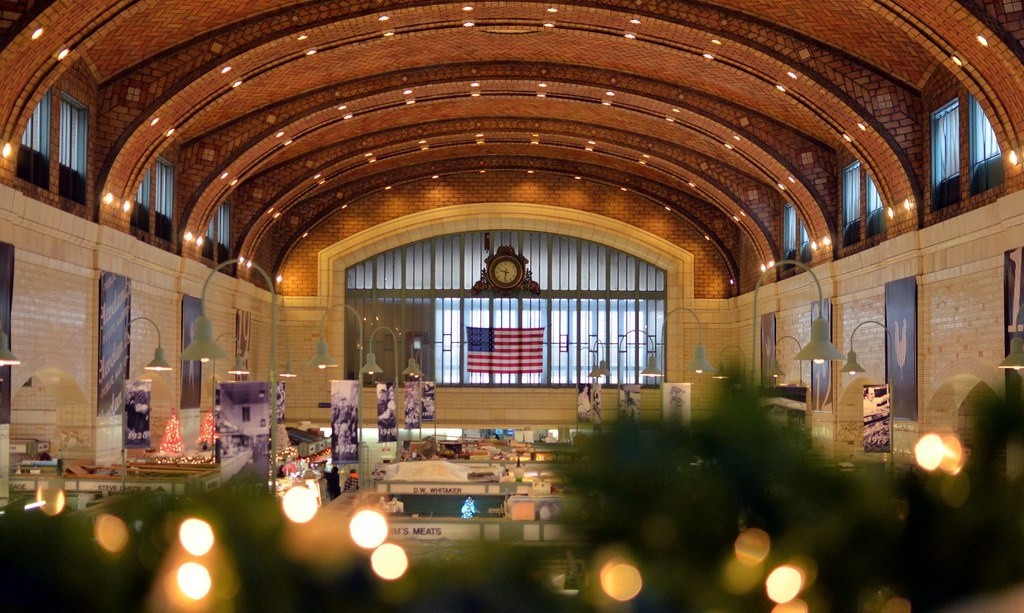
[619,329,663,417]
[663,306,717,420]
[179,258,297,490]
[306,303,364,492]
[997,303,1024,370]
[357,325,400,463]
[0,328,21,365]
[712,344,748,394]
[121,316,172,490]
[752,258,895,454]
[404,338,425,462]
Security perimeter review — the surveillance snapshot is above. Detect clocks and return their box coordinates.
[471,245,541,297]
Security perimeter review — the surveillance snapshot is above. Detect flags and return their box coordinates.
[466,326,545,373]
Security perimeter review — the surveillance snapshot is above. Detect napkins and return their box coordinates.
[574,338,611,436]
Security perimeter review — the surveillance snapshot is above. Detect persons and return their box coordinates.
[863,386,890,415]
[332,395,358,461]
[378,382,396,425]
[578,386,591,414]
[322,466,342,500]
[343,468,360,492]
[125,388,149,434]
